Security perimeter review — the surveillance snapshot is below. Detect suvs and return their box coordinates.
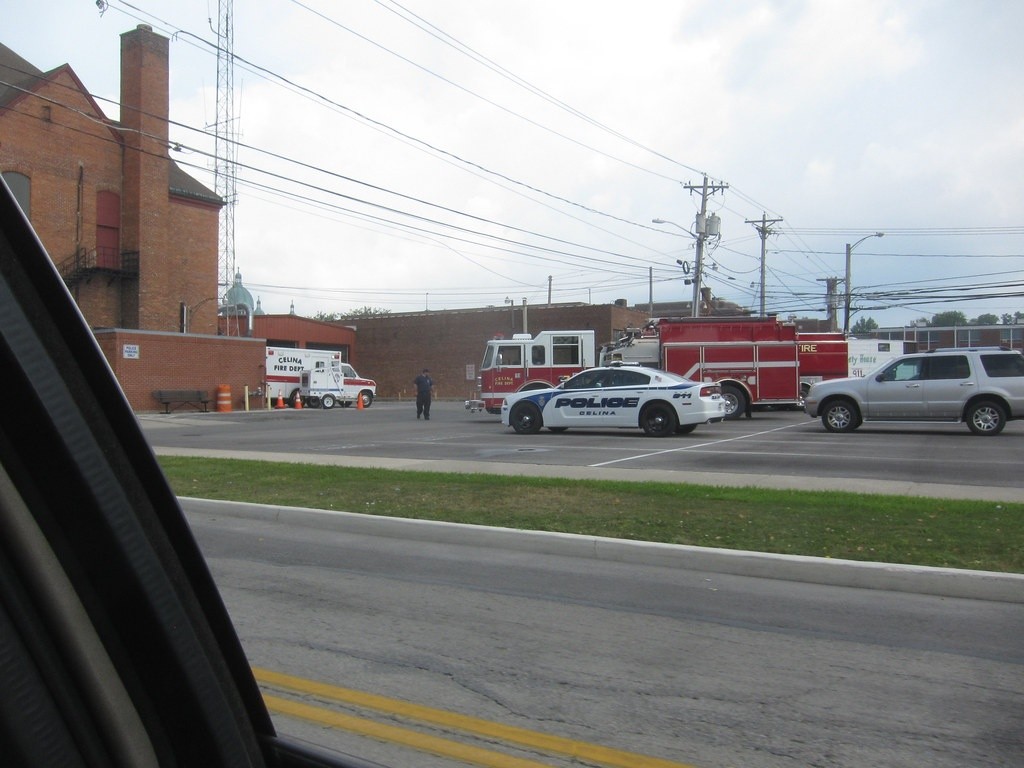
[802,346,1024,437]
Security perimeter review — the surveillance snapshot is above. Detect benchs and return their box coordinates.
[160,389,212,414]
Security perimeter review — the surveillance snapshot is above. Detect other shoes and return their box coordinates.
[425,416,429,420]
[416,414,421,418]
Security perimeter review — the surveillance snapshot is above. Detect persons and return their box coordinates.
[414,368,436,420]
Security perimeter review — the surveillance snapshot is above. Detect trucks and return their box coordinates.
[266,347,376,408]
[849,338,919,379]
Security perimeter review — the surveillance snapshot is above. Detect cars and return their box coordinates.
[500,362,727,438]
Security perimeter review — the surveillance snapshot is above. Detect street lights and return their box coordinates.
[181,295,228,333]
[845,232,883,333]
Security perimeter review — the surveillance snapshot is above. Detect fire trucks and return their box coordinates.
[463,315,801,420]
[795,333,850,411]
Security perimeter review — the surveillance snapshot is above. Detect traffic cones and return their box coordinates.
[293,390,303,408]
[274,389,285,409]
[356,392,363,409]
